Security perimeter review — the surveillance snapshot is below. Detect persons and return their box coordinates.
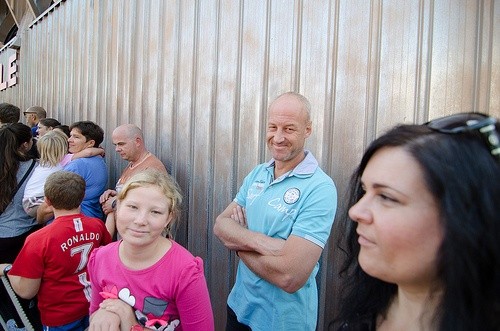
[99,124,168,215]
[213,92,337,331]
[0,122,40,264]
[0,169,116,331]
[0,103,71,159]
[327,112,500,331]
[37,121,109,227]
[85,167,214,331]
[22,128,105,217]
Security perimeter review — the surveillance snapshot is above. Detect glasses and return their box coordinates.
[24,111,37,115]
[424,112,500,161]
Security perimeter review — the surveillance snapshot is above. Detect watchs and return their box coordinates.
[3,264,13,278]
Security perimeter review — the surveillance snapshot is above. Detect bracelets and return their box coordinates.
[111,199,116,210]
